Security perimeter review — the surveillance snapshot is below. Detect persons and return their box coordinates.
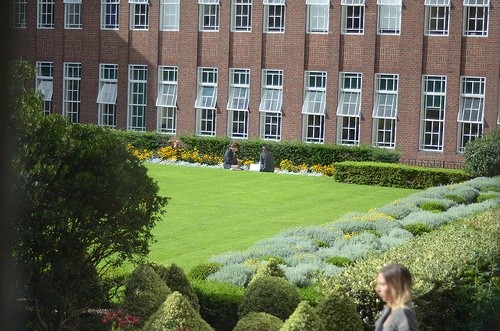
[258,145,275,172]
[372,263,421,330]
[222,143,243,171]
